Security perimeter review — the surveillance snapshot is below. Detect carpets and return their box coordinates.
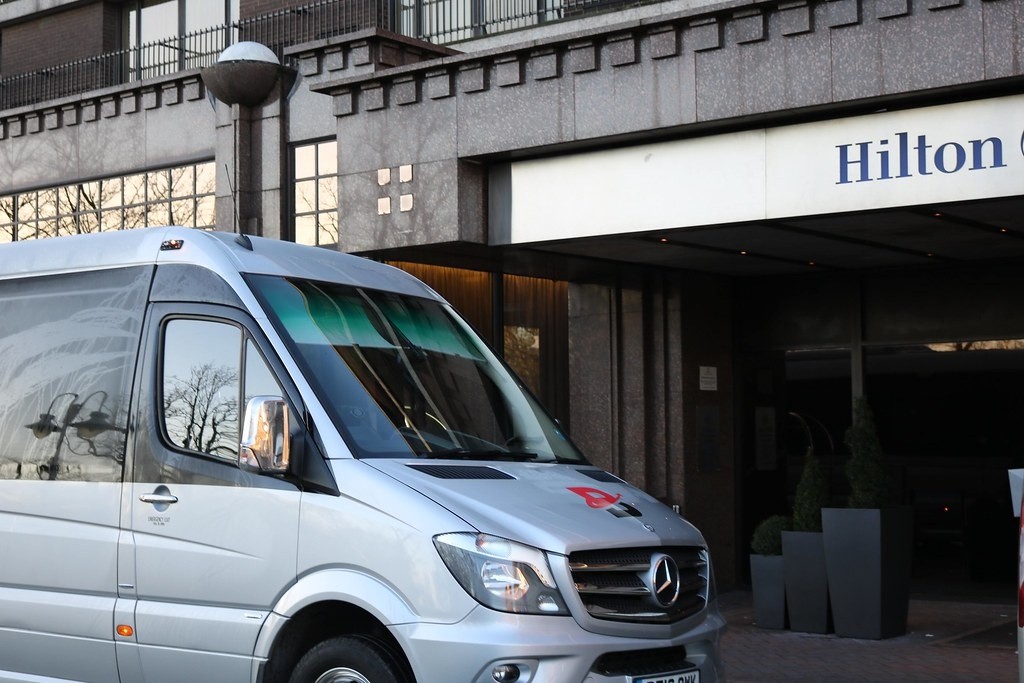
[931,617,1017,649]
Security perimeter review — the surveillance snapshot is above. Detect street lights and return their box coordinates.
[22,390,116,480]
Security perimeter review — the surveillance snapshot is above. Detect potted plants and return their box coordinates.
[751,395,914,641]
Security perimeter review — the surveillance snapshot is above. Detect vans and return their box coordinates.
[1,225,728,683]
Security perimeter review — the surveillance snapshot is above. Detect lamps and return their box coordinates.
[198,41,280,105]
